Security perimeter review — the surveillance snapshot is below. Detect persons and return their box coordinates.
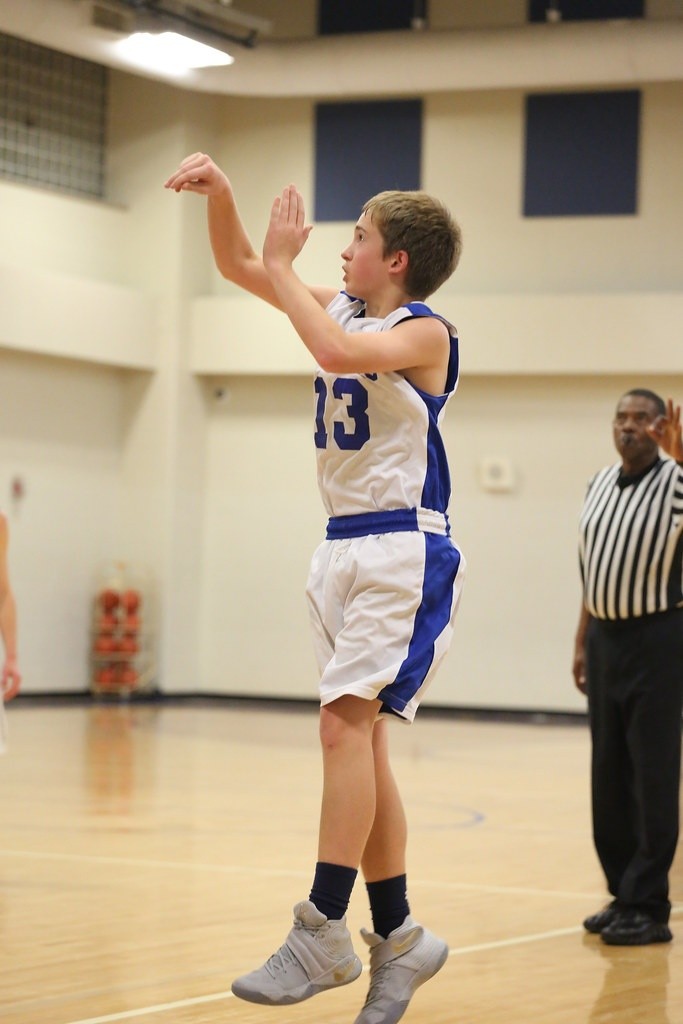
[164,150,464,1023]
[573,391,682,945]
[0,511,23,742]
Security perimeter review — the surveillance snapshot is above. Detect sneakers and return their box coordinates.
[599,907,673,947]
[229,900,362,1007]
[582,900,623,933]
[354,915,449,1023]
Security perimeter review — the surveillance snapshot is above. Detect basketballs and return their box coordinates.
[94,589,141,691]
